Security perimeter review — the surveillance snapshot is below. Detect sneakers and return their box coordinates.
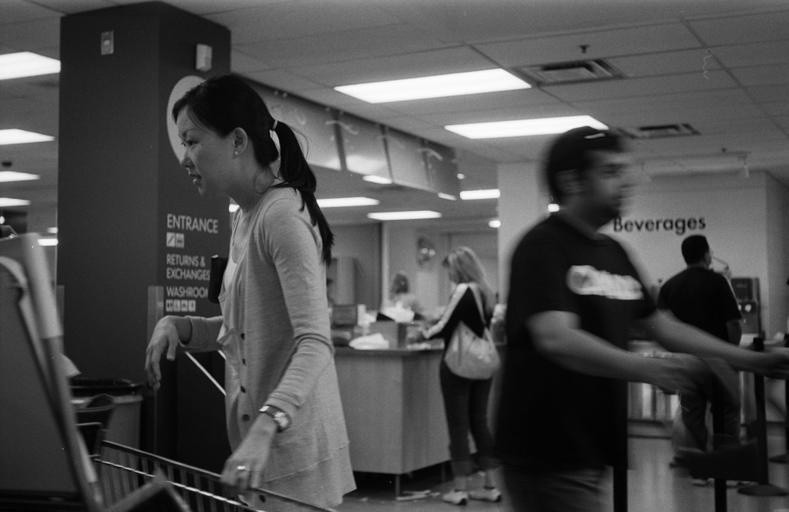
[468,487,502,503]
[440,488,469,506]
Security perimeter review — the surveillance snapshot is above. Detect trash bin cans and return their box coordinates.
[66,374,144,510]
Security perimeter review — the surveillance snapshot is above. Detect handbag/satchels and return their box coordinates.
[442,281,502,382]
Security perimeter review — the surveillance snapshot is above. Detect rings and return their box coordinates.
[235,464,248,472]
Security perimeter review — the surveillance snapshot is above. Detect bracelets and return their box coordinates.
[259,404,289,432]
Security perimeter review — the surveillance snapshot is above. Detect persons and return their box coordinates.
[660,233,757,490]
[144,70,359,511]
[415,247,502,505]
[490,127,787,512]
